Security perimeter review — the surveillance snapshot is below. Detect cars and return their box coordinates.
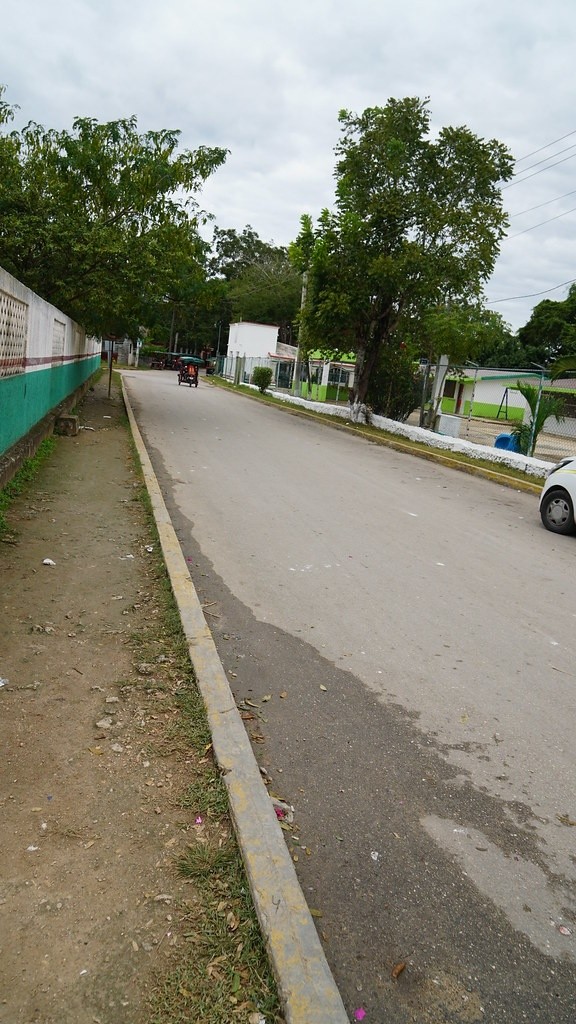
[537,455,576,532]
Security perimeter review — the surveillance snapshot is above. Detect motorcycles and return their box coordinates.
[164,352,192,370]
[177,357,205,388]
[151,352,172,370]
